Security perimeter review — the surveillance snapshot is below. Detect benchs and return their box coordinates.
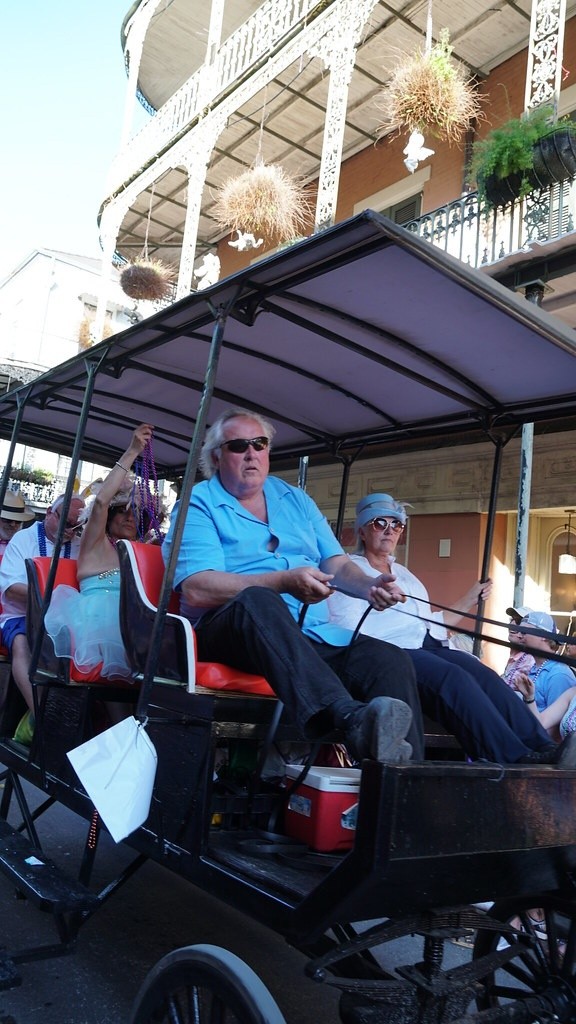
[0,529,505,748]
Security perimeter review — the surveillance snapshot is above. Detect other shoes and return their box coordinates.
[521,914,549,940]
[520,731,576,765]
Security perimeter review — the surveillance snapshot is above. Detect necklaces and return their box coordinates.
[38,521,71,558]
[88,809,98,850]
[534,658,550,684]
[507,651,527,687]
[106,429,166,551]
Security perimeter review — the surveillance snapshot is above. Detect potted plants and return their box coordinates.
[469,104,576,218]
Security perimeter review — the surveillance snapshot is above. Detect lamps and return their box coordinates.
[558,509,576,574]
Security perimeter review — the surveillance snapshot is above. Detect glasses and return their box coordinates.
[0,517,22,524]
[510,618,522,625]
[55,509,80,531]
[364,517,405,535]
[217,437,268,453]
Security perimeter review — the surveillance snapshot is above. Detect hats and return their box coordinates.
[0,490,35,521]
[90,473,171,529]
[356,493,416,528]
[506,606,533,618]
[522,611,564,645]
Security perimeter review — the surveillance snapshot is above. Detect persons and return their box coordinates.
[447,605,576,955]
[323,492,576,770]
[45,424,155,683]
[0,494,133,725]
[161,408,426,769]
[0,491,35,556]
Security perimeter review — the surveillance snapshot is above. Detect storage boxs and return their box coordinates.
[282,763,367,849]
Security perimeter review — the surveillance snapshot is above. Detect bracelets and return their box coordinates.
[523,698,535,704]
[115,462,130,472]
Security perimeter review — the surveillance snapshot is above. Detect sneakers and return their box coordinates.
[344,696,413,762]
